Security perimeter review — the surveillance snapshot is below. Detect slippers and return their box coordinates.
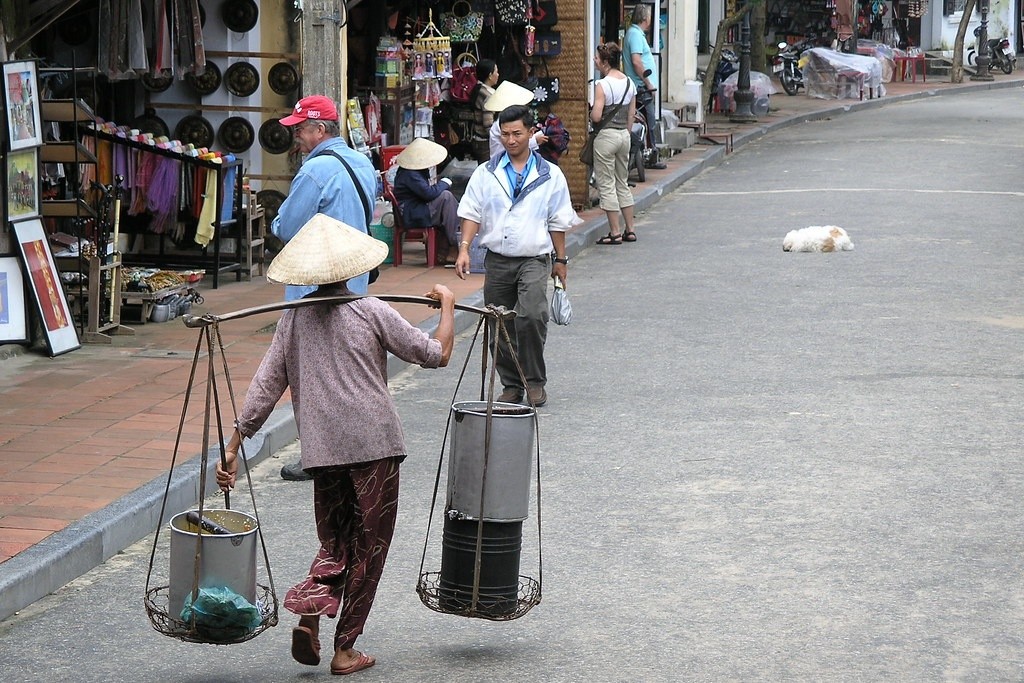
[330,648,376,674]
[291,627,321,666]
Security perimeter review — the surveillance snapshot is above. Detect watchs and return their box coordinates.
[553,255,570,264]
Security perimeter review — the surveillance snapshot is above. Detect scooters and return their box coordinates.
[589,69,653,187]
[699,45,741,103]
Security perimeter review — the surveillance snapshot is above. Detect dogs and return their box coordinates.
[782,225,854,252]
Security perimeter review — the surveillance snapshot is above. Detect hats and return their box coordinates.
[279,94,340,126]
[484,80,534,111]
[396,137,448,169]
[266,212,390,284]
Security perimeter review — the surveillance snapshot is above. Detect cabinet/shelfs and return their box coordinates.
[35,49,104,325]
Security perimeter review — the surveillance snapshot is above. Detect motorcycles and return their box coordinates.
[770,31,816,95]
[967,25,1017,74]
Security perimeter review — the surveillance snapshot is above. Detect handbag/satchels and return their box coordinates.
[368,270,379,285]
[525,55,560,104]
[579,133,597,165]
[438,0,485,44]
[448,53,479,102]
[495,0,562,55]
[447,103,476,142]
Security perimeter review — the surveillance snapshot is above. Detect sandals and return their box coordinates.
[622,230,637,242]
[595,232,622,244]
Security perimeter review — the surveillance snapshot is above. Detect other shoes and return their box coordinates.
[645,162,667,169]
[526,386,547,406]
[497,387,524,403]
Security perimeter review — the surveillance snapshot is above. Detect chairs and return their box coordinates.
[386,185,436,268]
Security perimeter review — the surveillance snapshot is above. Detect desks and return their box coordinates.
[833,70,881,102]
[894,57,925,84]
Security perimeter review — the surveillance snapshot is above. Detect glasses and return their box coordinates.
[290,127,302,133]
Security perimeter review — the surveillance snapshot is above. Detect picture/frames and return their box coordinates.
[8,214,82,359]
[0,58,46,151]
[0,251,34,346]
[3,147,43,233]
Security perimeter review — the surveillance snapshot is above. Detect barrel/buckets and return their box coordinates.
[445,400,535,522]
[438,515,523,618]
[167,508,259,635]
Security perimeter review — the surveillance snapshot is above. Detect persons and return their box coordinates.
[589,41,637,246]
[622,3,667,170]
[270,94,377,483]
[391,137,460,266]
[468,58,549,164]
[213,211,456,676]
[453,104,585,406]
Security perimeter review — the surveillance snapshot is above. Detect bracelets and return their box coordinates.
[458,239,470,246]
